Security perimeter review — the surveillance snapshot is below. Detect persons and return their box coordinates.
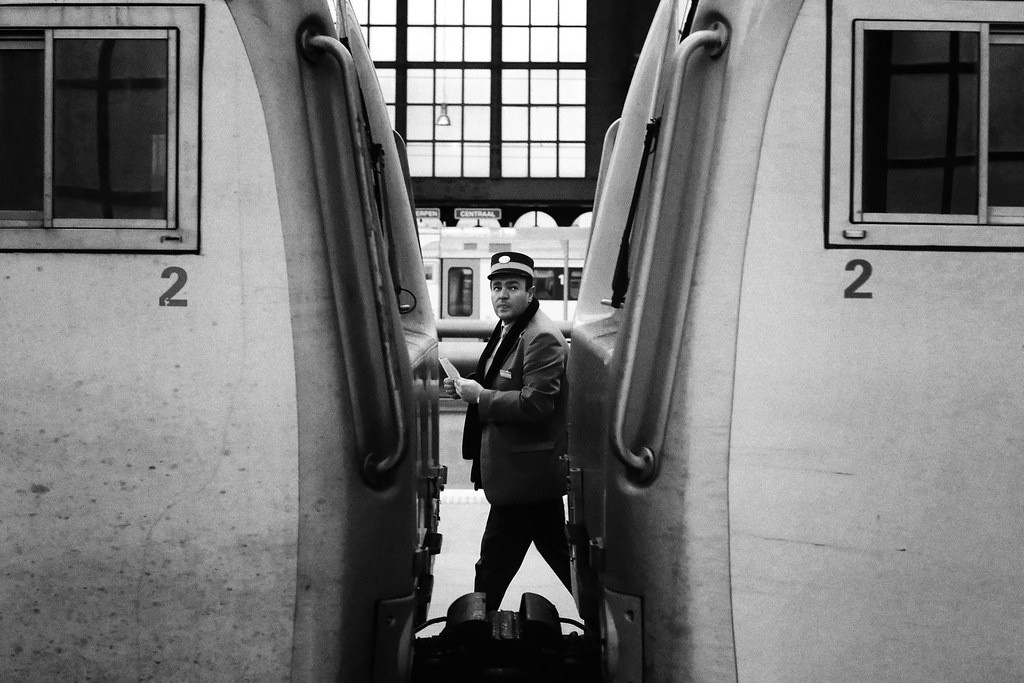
[444,252,574,615]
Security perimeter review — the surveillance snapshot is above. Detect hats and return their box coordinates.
[487,252,534,280]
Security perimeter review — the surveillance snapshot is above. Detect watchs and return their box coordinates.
[476,389,484,404]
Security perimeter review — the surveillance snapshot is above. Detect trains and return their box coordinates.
[0,0,1024,683]
[414,228,590,343]
